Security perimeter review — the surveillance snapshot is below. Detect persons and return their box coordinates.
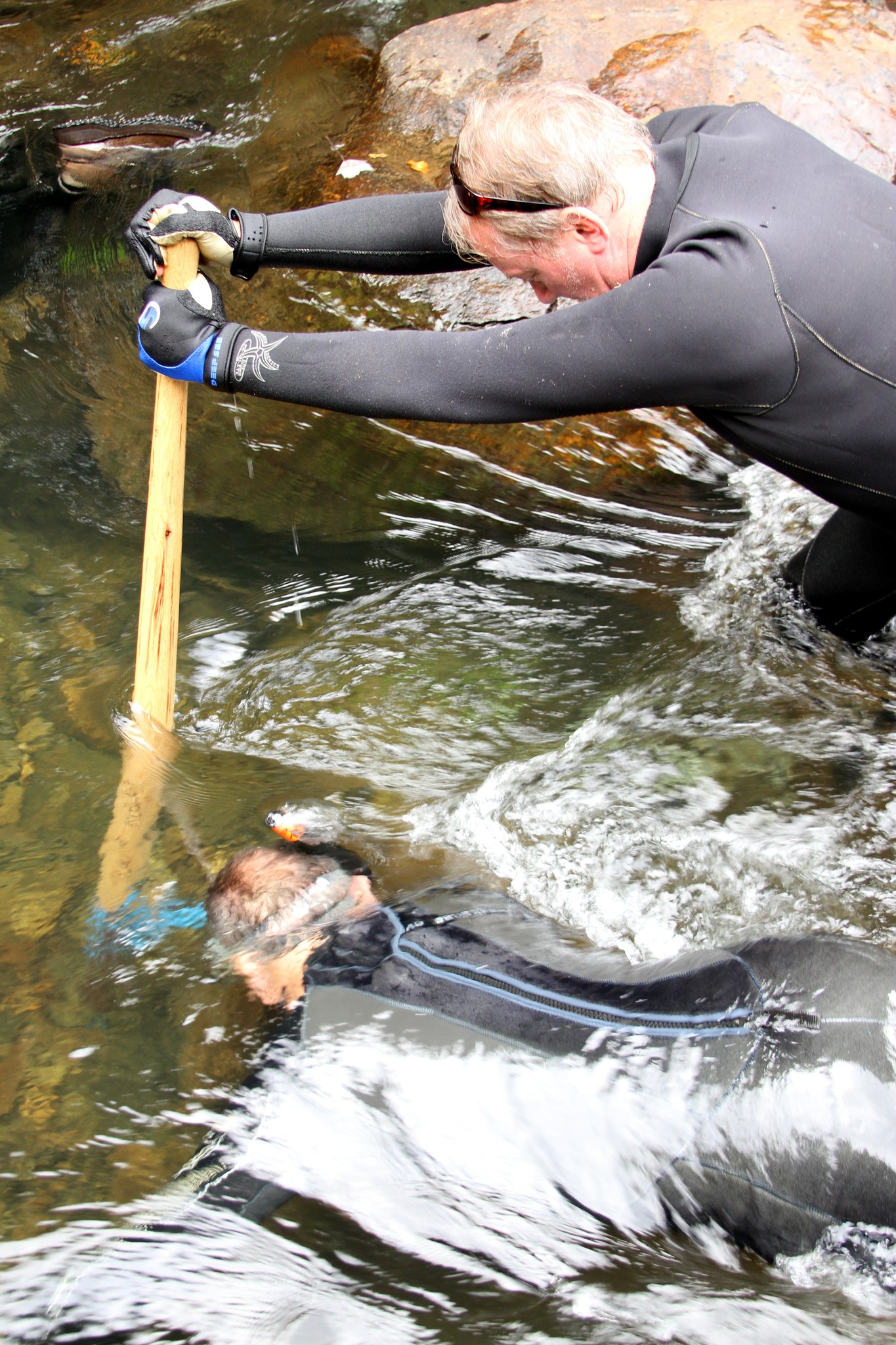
[0,833,896,1345]
[123,78,896,647]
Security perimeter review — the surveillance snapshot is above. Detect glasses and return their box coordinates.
[449,136,573,216]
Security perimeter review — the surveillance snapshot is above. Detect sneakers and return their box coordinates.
[52,117,215,178]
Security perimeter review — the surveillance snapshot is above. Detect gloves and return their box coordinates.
[124,189,267,281]
[136,265,249,394]
